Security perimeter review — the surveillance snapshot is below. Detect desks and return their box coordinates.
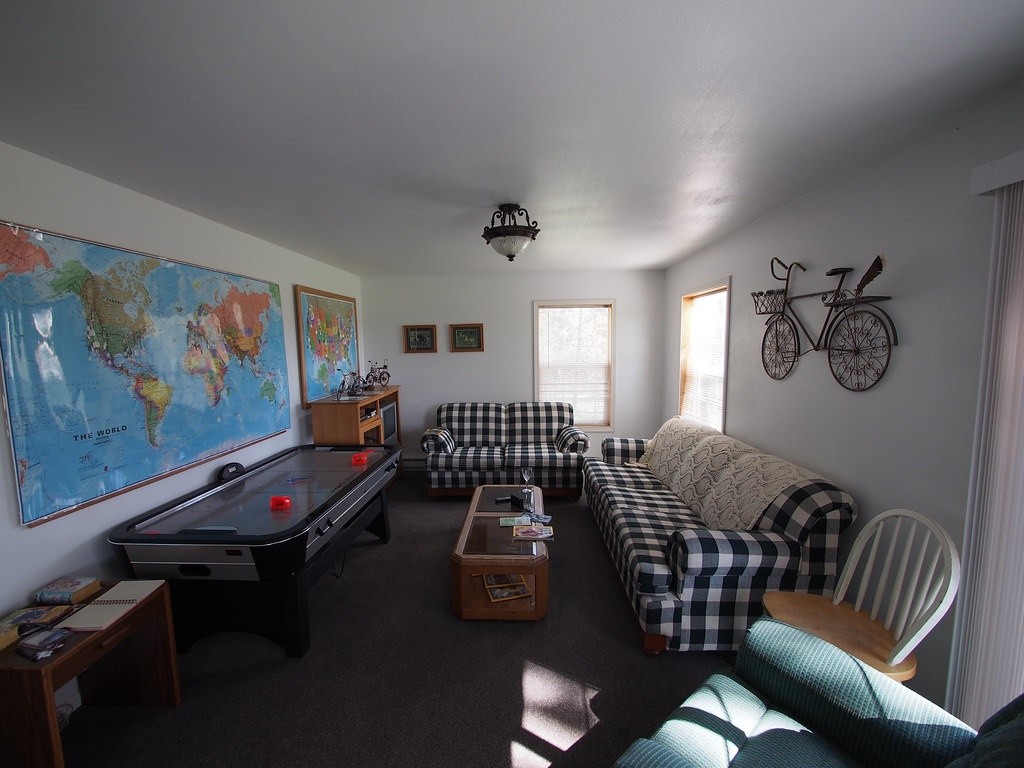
[106,443,403,658]
[0,580,181,768]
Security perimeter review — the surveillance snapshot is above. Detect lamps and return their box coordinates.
[481,203,541,261]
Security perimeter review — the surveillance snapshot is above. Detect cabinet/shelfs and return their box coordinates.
[309,385,404,491]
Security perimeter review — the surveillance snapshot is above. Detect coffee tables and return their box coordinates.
[449,484,550,621]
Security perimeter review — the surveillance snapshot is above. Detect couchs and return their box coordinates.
[420,402,589,502]
[614,618,1024,768]
[583,414,858,658]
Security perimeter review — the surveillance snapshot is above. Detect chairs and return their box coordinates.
[762,508,960,685]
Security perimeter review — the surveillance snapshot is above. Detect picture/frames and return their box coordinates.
[449,324,485,352]
[401,325,437,353]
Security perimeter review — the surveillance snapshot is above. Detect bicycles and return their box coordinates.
[336,369,366,401]
[366,359,391,389]
[750,254,899,392]
